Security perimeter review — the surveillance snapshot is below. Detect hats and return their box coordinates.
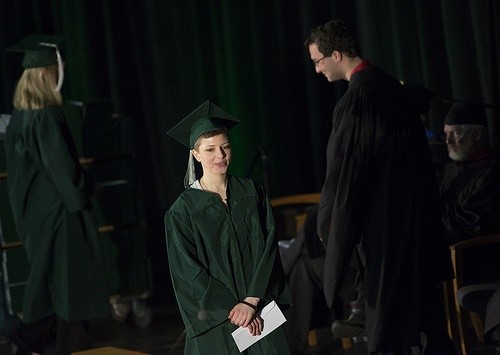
[5,33,75,92]
[166,100,241,189]
[442,96,499,125]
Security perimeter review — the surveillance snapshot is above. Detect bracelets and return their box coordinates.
[242,300,256,310]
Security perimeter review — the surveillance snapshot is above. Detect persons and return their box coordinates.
[305,21,451,355]
[6,34,120,323]
[332,101,500,355]
[164,100,290,355]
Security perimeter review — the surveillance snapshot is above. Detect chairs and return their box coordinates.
[269,194,500,355]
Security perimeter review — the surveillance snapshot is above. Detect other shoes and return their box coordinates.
[109,293,130,322]
[331,301,368,343]
[130,292,151,329]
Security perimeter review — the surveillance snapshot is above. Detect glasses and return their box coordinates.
[312,47,344,67]
